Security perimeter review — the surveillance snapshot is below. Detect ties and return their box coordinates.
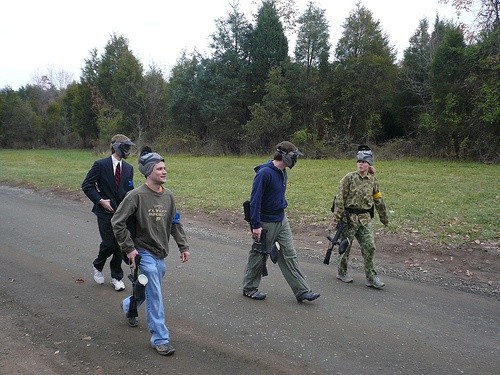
[114,162,120,194]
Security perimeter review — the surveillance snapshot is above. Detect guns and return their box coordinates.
[125,254,148,319]
[244,200,281,277]
[106,199,119,212]
[323,221,351,264]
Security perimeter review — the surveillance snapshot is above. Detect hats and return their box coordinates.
[356,144,374,165]
[138,146,165,177]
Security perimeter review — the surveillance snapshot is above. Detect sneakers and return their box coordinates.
[366,277,385,288]
[244,290,266,300]
[297,291,320,302]
[121,301,139,326]
[112,277,126,291]
[337,273,354,283]
[151,342,176,355]
[92,264,104,284]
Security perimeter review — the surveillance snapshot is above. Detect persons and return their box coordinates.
[110,150,191,356]
[332,145,391,288]
[81,133,136,291]
[240,141,321,302]
